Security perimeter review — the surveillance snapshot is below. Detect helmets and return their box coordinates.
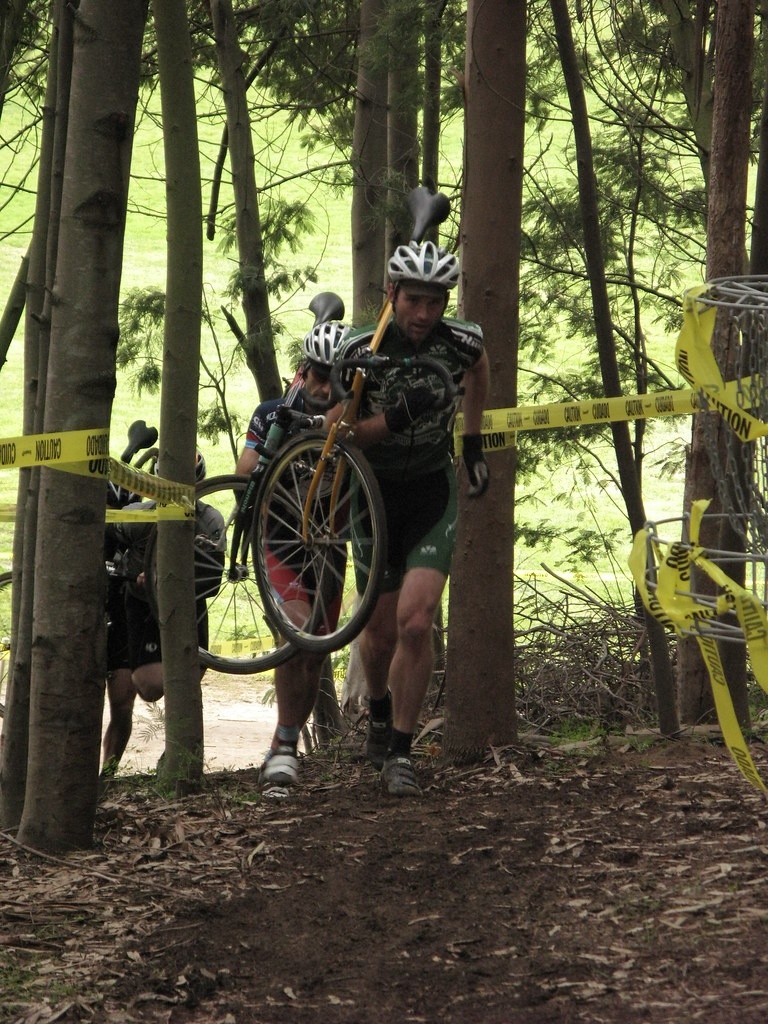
[387,240,459,289]
[303,321,356,366]
[154,447,206,482]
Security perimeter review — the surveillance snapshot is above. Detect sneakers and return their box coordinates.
[367,691,391,772]
[381,753,419,797]
[257,745,300,790]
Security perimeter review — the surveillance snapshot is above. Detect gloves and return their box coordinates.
[385,386,438,434]
[462,434,490,499]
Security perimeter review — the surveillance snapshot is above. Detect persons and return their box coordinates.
[234,322,368,787]
[323,238,492,799]
[100,448,226,779]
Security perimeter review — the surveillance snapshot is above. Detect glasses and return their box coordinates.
[312,368,329,383]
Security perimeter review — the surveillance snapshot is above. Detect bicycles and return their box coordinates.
[0,419,160,724]
[245,186,452,654]
[143,290,347,678]
